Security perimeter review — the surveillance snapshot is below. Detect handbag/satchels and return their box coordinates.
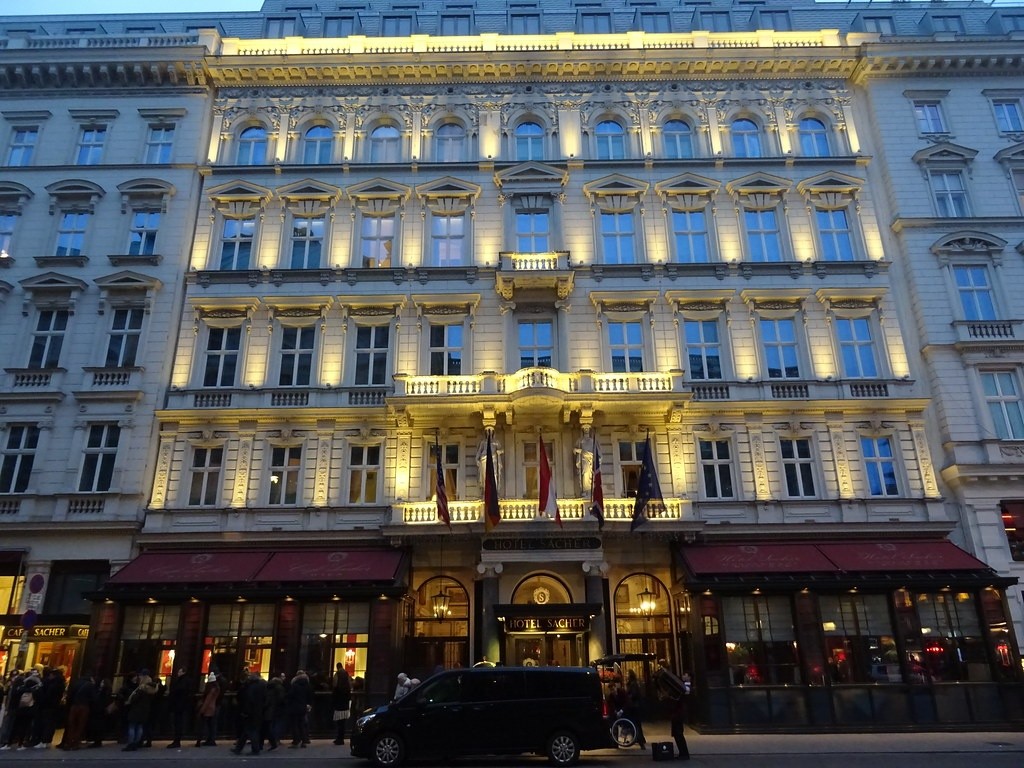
[652,741,674,761]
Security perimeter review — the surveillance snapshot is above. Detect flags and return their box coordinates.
[590,443,608,532]
[538,437,563,529]
[437,441,452,531]
[483,439,501,536]
[630,439,666,532]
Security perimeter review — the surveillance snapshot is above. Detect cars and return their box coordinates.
[743,644,802,685]
[871,641,941,684]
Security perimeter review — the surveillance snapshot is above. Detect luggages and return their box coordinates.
[654,668,685,701]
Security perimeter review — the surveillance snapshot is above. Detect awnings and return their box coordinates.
[107,549,407,583]
[679,539,992,574]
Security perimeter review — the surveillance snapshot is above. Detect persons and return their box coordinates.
[476,427,505,498]
[496,662,505,667]
[627,670,643,703]
[573,425,603,496]
[608,682,646,750]
[646,671,693,760]
[394,673,421,698]
[0,669,354,756]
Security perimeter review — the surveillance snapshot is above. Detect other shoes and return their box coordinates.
[0,744,11,750]
[17,746,26,750]
[333,739,344,745]
[56,740,311,755]
[34,742,51,749]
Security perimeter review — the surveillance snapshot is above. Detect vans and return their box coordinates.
[348,651,659,768]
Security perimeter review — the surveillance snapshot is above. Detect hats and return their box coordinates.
[138,668,150,676]
[208,671,216,682]
